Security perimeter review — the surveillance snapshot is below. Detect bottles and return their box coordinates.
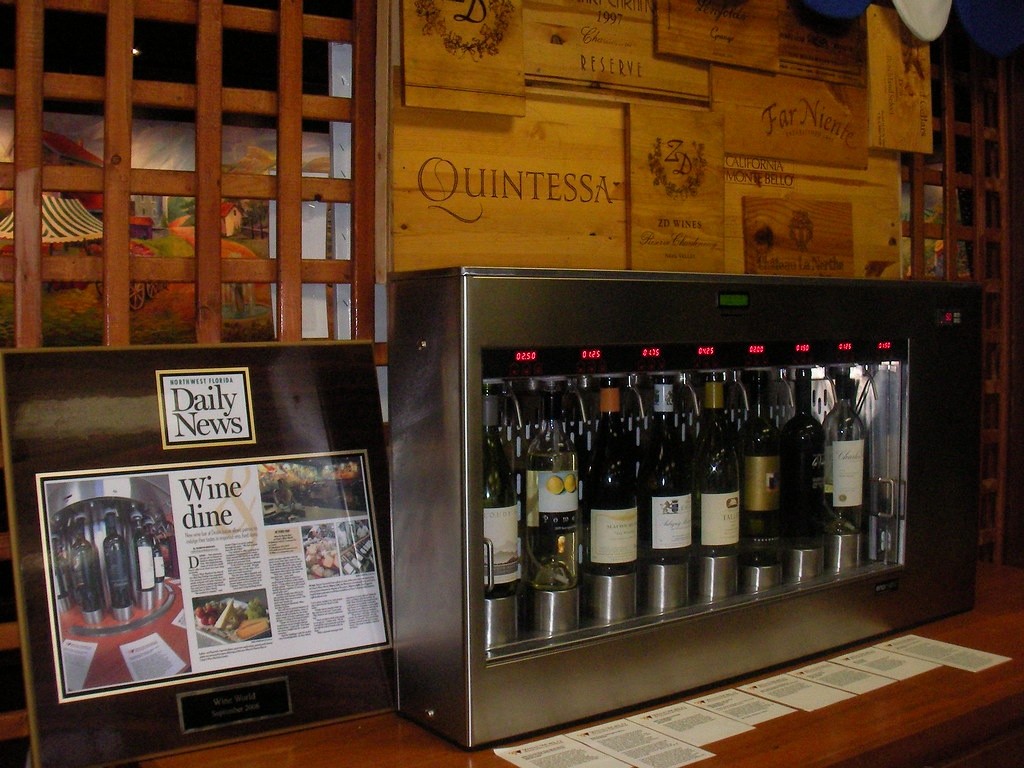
[691,372,737,557]
[71,517,101,611]
[130,520,155,591]
[152,536,166,582]
[523,384,579,592]
[640,375,692,563]
[481,379,517,598]
[583,377,637,574]
[737,371,783,567]
[824,380,869,535]
[103,512,133,609]
[781,368,824,550]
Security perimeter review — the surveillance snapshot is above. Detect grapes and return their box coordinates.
[243,599,266,620]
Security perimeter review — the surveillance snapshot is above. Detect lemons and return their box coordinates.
[547,477,564,494]
[564,474,576,493]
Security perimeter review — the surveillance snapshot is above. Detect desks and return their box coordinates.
[137,561,1023,768]
